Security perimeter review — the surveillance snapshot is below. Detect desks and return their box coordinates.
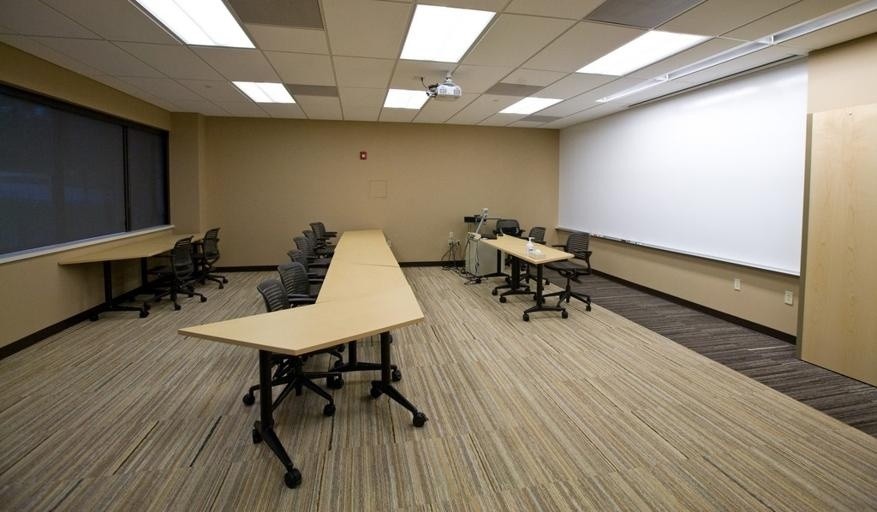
[57,230,206,321]
[177,229,428,489]
[479,232,575,321]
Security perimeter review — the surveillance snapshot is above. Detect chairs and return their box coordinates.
[191,228,228,289]
[242,222,345,417]
[542,231,592,312]
[143,235,207,310]
[505,226,550,293]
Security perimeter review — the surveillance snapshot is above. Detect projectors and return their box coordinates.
[435,81,461,97]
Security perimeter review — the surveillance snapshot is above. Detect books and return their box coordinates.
[526,247,547,259]
